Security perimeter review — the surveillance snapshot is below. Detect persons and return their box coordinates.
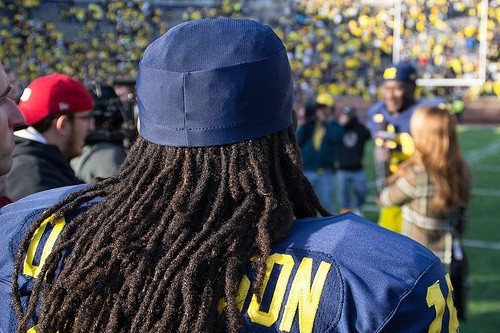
[0,19,459,333]
[373,105,471,323]
[0,62,26,208]
[0,1,500,111]
[70,78,137,184]
[366,62,447,236]
[1,73,95,202]
[295,91,372,219]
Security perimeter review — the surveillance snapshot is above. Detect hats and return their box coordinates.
[382,61,416,82]
[86,83,117,102]
[14,73,94,123]
[136,17,293,147]
[316,93,334,106]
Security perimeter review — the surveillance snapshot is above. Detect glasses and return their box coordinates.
[72,111,94,121]
